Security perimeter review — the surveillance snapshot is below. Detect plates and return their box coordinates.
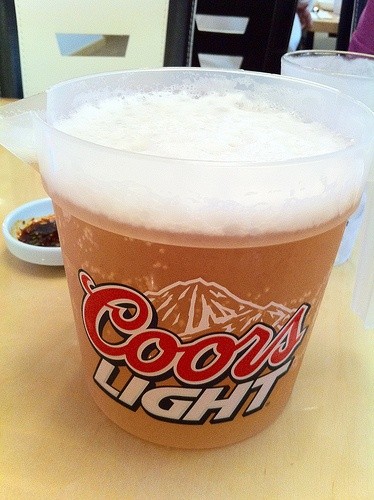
[4,198,64,265]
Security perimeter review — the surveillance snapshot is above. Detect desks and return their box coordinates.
[296,1,341,51]
[0,98,374,500]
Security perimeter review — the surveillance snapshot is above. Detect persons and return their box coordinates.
[192,0,315,75]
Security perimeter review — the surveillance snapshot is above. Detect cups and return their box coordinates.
[281,50,374,266]
[1,68,374,448]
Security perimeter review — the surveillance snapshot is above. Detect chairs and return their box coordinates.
[0,0,197,100]
[191,0,298,75]
